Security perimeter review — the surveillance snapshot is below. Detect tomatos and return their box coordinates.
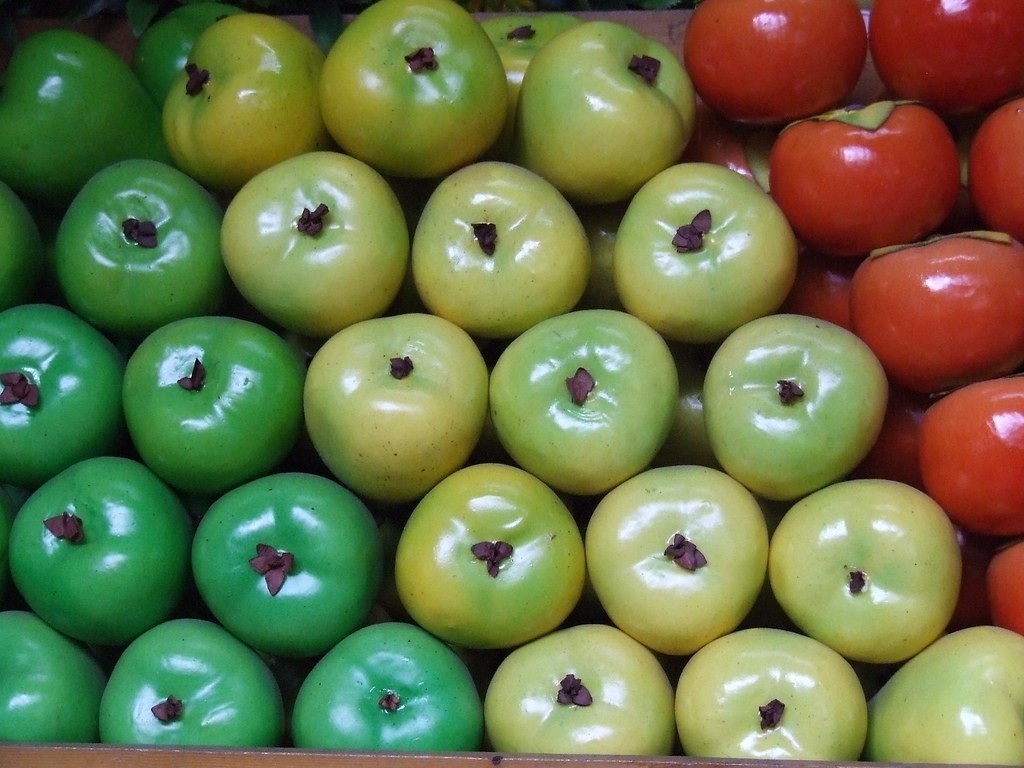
[0,0,1023,768]
